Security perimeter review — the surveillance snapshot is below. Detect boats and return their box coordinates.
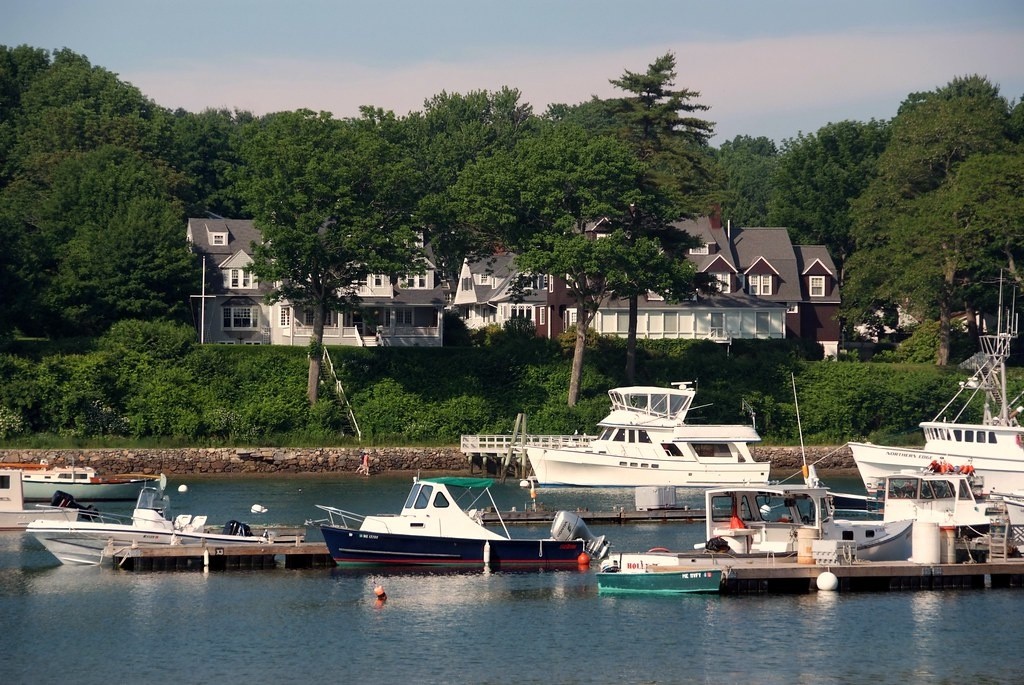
[24,473,270,567]
[314,477,613,566]
[523,382,772,489]
[593,267,1024,592]
[203,524,308,544]
[1,452,167,533]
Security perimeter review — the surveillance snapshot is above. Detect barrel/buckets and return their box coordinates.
[939,525,957,564]
[911,522,941,564]
[795,528,820,564]
